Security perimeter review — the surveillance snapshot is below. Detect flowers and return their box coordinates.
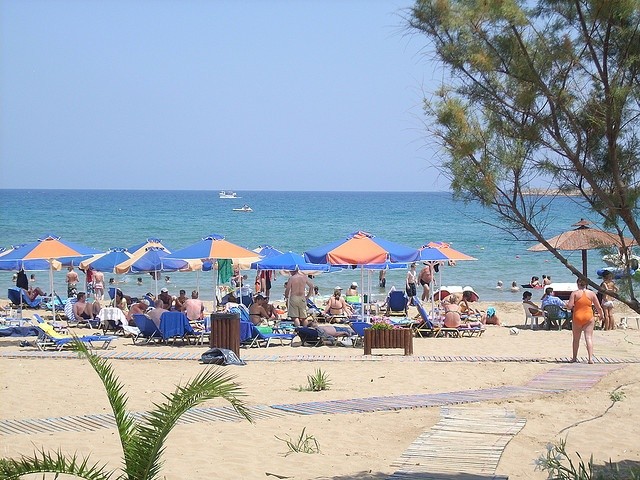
[370,316,403,329]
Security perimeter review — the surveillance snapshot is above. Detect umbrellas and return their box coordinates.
[113,246,190,299]
[526,218,639,289]
[0,232,105,325]
[159,233,267,312]
[126,237,174,292]
[303,230,422,323]
[250,250,331,273]
[416,241,479,322]
[77,247,146,308]
[0,243,63,310]
[251,244,285,270]
[332,261,408,316]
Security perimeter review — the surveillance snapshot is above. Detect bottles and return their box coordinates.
[17,306,22,320]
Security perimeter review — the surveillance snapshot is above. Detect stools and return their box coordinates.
[620,317,640,329]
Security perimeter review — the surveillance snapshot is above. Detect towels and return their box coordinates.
[159,312,194,340]
[239,322,253,342]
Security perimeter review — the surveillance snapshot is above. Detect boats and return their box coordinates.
[232,205,252,211]
[219,190,237,198]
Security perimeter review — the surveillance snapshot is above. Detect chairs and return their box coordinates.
[523,302,545,329]
[132,314,163,345]
[159,312,211,346]
[239,322,295,348]
[412,296,485,338]
[7,287,51,310]
[143,296,154,306]
[33,313,45,324]
[1,315,31,325]
[386,291,409,317]
[100,308,130,335]
[65,298,99,329]
[297,325,358,347]
[350,322,372,345]
[544,305,571,331]
[307,297,358,323]
[109,287,137,308]
[29,321,117,351]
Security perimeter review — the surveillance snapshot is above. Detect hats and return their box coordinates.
[334,286,343,290]
[351,282,359,287]
[161,288,168,292]
[463,285,479,301]
[140,299,150,306]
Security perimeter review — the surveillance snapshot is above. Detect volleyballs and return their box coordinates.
[510,327,519,336]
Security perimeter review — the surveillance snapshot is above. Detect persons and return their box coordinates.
[127,299,150,327]
[447,260,456,266]
[111,289,130,317]
[284,267,314,326]
[249,295,269,326]
[146,299,170,332]
[321,289,352,322]
[475,306,501,326]
[418,265,435,301]
[379,270,386,287]
[73,292,101,320]
[547,276,552,284]
[282,282,289,310]
[600,271,619,331]
[314,286,320,296]
[346,282,359,296]
[495,281,503,289]
[307,321,351,337]
[65,266,80,298]
[564,278,604,364]
[93,269,106,300]
[15,268,28,291]
[174,290,188,312]
[541,274,549,288]
[532,276,540,289]
[522,291,542,316]
[405,264,418,305]
[150,272,155,280]
[529,276,536,288]
[85,268,94,298]
[597,269,610,330]
[511,281,520,292]
[440,290,472,316]
[540,287,572,329]
[223,296,239,314]
[261,295,278,321]
[29,274,36,282]
[11,274,17,282]
[20,286,47,302]
[164,276,171,283]
[180,290,204,321]
[109,277,116,284]
[327,286,354,310]
[158,287,172,309]
[444,295,462,338]
[136,277,143,285]
[230,269,245,287]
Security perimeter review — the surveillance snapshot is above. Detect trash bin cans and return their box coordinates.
[211,313,239,360]
